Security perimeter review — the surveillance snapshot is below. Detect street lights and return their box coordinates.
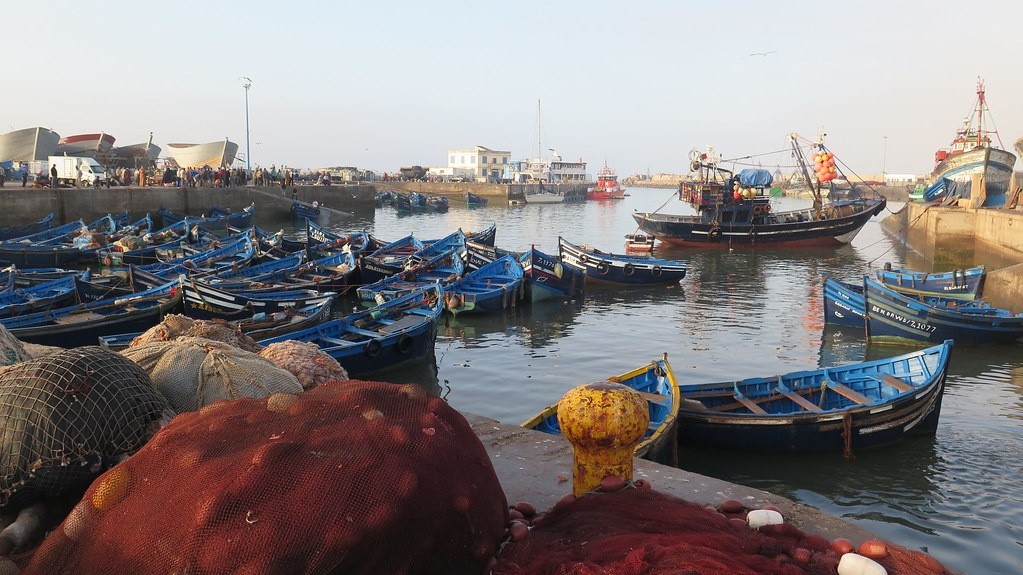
[883,136,887,181]
[242,77,252,180]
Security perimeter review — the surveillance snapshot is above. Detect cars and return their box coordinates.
[449,174,465,183]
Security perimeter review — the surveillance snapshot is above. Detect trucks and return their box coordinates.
[30,156,106,187]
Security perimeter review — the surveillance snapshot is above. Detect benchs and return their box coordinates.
[322,308,433,346]
[387,269,455,290]
[734,372,913,416]
[456,281,505,295]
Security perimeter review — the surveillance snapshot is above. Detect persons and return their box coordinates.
[104,166,333,188]
[281,180,286,197]
[51,164,57,190]
[75,165,83,191]
[20,163,29,186]
[785,214,804,223]
[31,170,48,187]
[292,187,298,201]
[0,165,6,187]
[383,171,394,181]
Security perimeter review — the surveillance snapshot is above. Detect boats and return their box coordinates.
[923,76,1017,207]
[443,253,526,315]
[519,352,681,461]
[876,262,987,301]
[0,126,162,162]
[822,272,1023,343]
[558,236,687,284]
[518,244,586,302]
[0,201,526,352]
[624,235,655,253]
[678,340,954,450]
[525,99,631,203]
[466,192,488,208]
[167,138,239,169]
[632,127,886,248]
[256,278,445,371]
[375,191,449,211]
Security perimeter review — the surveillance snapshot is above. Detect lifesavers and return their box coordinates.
[580,254,588,263]
[392,308,403,320]
[367,339,383,358]
[651,266,663,277]
[708,229,722,241]
[764,205,771,212]
[596,261,609,274]
[753,206,761,214]
[622,263,635,276]
[563,177,568,184]
[398,335,415,354]
